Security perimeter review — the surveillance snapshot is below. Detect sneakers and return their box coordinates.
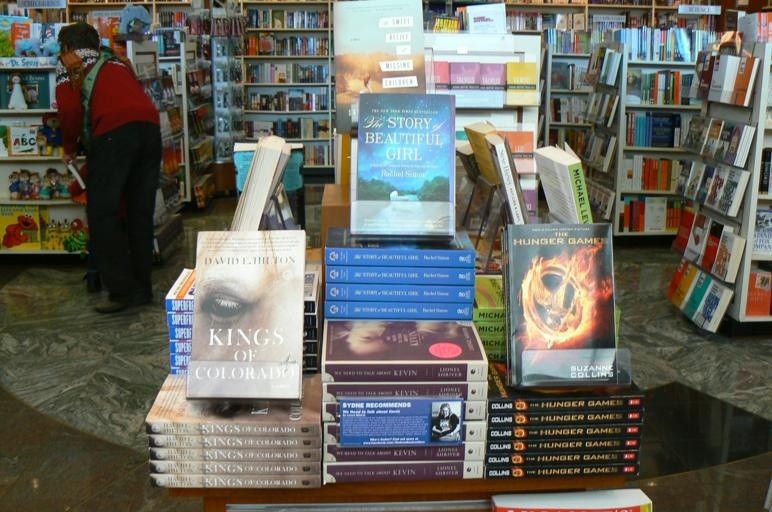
[95,289,152,313]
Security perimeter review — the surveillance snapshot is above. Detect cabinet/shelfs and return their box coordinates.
[1,2,209,266]
[576,0,772,321]
[205,0,718,169]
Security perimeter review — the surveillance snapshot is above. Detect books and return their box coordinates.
[7,125,40,155]
[3,206,40,251]
[653,1,771,332]
[145,1,652,512]
[3,1,161,82]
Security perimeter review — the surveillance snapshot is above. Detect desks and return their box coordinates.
[167,475,630,512]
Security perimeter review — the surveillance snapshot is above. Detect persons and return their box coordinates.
[9,169,75,200]
[42,113,64,157]
[8,73,27,109]
[57,22,161,312]
